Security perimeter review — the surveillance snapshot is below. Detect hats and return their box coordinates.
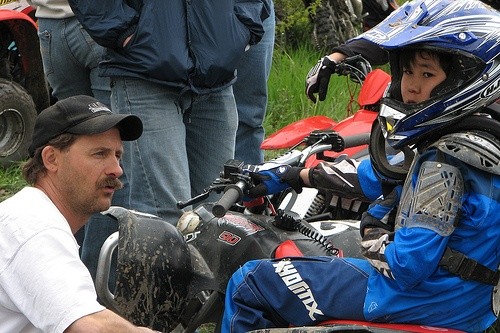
[28,95,143,158]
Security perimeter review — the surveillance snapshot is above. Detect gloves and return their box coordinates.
[304,55,336,104]
[242,165,305,198]
[360,183,402,237]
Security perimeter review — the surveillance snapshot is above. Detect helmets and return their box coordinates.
[362,0,500,149]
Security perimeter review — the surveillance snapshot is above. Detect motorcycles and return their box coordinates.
[82,54,500,333]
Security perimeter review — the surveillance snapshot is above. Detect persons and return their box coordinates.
[26,0,500,333]
[0,95,163,333]
